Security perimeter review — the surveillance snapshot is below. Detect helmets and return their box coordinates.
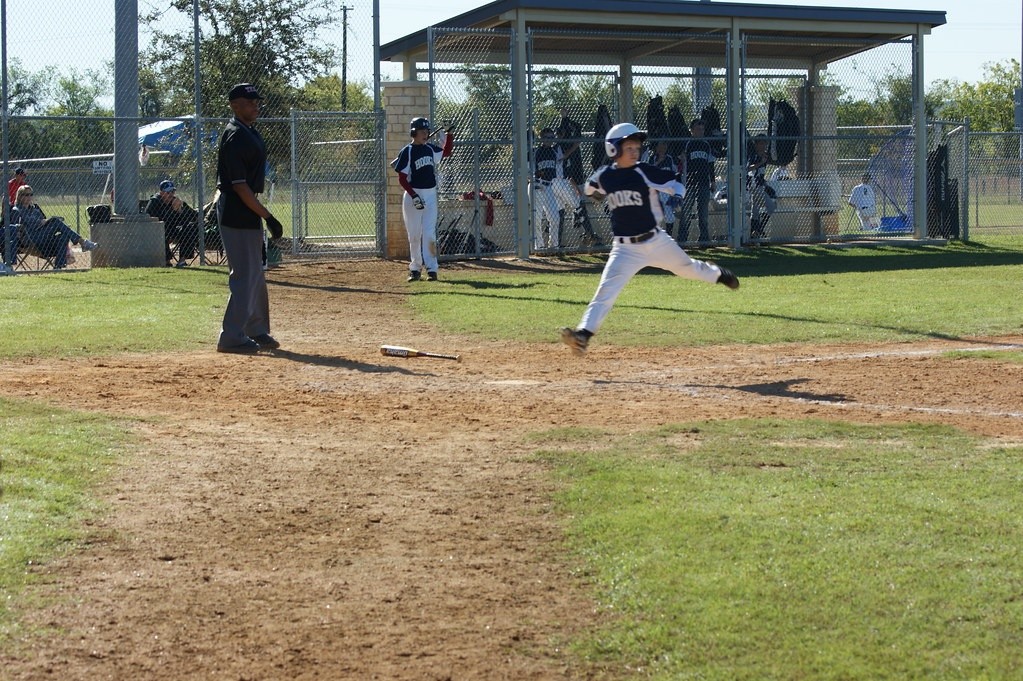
[605,123,647,159]
[411,117,431,130]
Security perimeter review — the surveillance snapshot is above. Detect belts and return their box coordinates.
[620,228,659,243]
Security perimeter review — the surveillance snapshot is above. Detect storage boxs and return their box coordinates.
[880,215,906,232]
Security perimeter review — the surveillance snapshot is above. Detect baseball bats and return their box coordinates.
[390,120,451,168]
[380,345,463,363]
[461,209,478,254]
[436,214,462,253]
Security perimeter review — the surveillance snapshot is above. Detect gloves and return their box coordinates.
[665,194,683,208]
[412,194,424,210]
[441,119,455,134]
[264,214,283,239]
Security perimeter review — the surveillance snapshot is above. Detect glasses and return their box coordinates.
[249,98,258,105]
[543,136,554,139]
[21,193,33,196]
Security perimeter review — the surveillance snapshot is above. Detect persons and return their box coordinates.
[560,123,740,358]
[647,118,788,246]
[11,185,99,270]
[144,180,195,268]
[216,83,283,354]
[527,128,605,257]
[8,167,27,205]
[395,117,454,283]
[848,174,878,231]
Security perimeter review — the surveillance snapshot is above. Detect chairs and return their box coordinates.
[9,202,72,277]
[139,200,200,267]
[195,224,228,266]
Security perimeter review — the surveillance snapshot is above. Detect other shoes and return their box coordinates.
[250,333,279,348]
[706,260,739,289]
[427,272,437,280]
[218,338,260,353]
[81,238,98,252]
[560,327,590,357]
[408,271,421,282]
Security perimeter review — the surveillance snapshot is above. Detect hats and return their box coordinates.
[160,180,176,191]
[16,168,24,173]
[229,84,265,100]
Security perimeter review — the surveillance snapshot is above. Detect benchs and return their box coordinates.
[500,180,841,246]
